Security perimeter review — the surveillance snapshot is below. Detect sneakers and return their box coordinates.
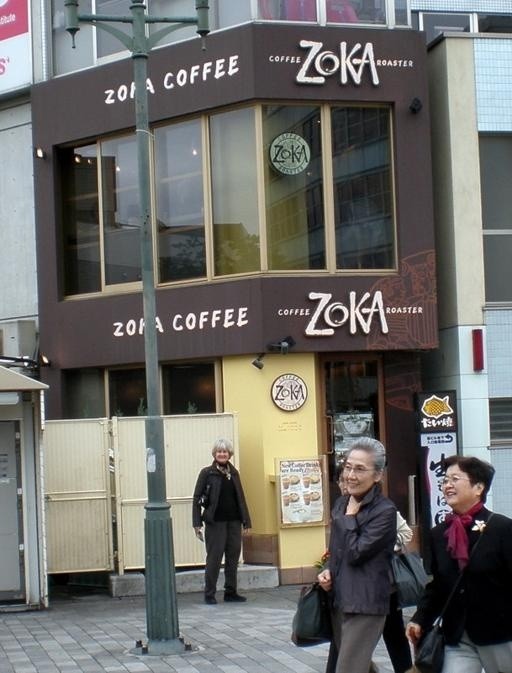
[205,596,217,604]
[224,592,247,602]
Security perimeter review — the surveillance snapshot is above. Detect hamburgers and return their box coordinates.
[288,474,320,502]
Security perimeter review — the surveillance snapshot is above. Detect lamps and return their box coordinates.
[273,336,295,353]
[408,98,423,113]
[252,353,266,369]
[34,146,48,161]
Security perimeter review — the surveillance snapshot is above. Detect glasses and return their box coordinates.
[442,474,469,487]
[341,462,378,475]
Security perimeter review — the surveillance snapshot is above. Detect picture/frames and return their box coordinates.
[274,455,329,527]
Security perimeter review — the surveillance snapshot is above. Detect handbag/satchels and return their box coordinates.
[414,614,445,673]
[290,584,332,648]
[390,549,429,608]
[196,494,209,509]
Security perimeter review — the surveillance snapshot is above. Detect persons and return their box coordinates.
[191,438,252,605]
[404,454,511,673]
[320,455,414,673]
[315,436,397,673]
